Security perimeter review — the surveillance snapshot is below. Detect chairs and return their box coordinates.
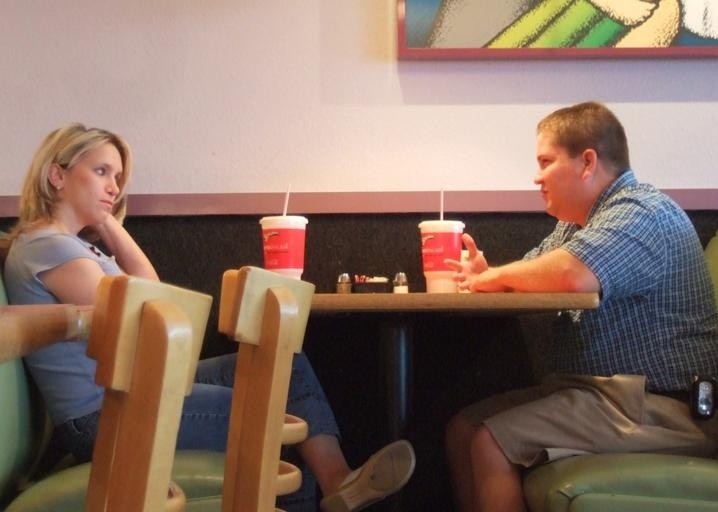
[84,273,213,512]
[218,266,316,512]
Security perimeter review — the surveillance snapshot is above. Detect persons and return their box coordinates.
[443,101,717,511]
[4,125,416,511]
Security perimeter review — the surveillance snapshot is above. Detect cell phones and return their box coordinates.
[698,382,711,416]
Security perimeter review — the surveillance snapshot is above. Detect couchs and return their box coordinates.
[522,223,718,512]
[0,229,227,512]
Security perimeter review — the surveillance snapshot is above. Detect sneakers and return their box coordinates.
[318,439,415,511]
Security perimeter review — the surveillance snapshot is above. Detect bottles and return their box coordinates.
[393,272,409,294]
[336,273,352,293]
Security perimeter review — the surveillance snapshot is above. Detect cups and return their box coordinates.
[259,215,309,281]
[417,219,467,294]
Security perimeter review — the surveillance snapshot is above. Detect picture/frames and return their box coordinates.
[395,0,718,62]
